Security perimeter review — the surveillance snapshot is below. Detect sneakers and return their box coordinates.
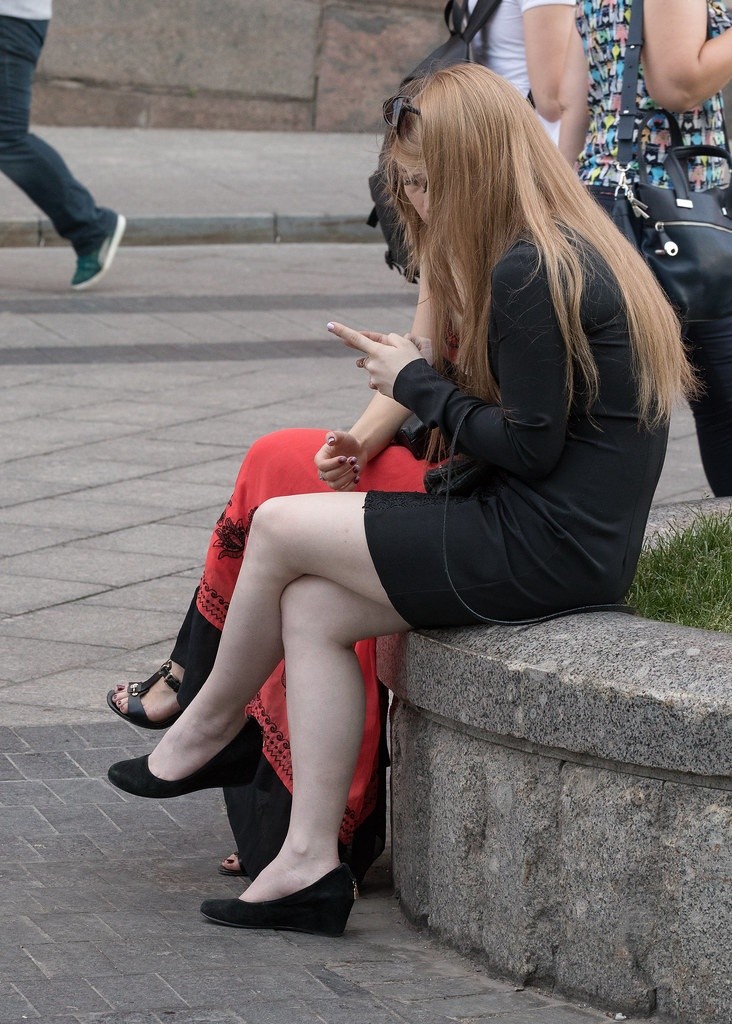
[70,209,126,291]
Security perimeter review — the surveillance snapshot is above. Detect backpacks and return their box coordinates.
[366,0,502,285]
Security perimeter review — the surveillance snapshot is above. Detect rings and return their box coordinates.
[319,471,326,481]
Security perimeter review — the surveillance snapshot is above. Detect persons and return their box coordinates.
[107,1,732,937]
[1,1,128,292]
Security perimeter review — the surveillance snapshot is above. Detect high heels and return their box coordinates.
[107,715,262,799]
[200,863,359,938]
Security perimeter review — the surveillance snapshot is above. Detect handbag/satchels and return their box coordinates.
[423,457,487,496]
[396,413,430,460]
[626,109,732,323]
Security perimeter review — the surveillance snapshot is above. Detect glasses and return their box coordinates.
[382,95,420,134]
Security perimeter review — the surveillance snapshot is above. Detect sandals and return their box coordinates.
[107,659,181,729]
[217,851,240,876]
